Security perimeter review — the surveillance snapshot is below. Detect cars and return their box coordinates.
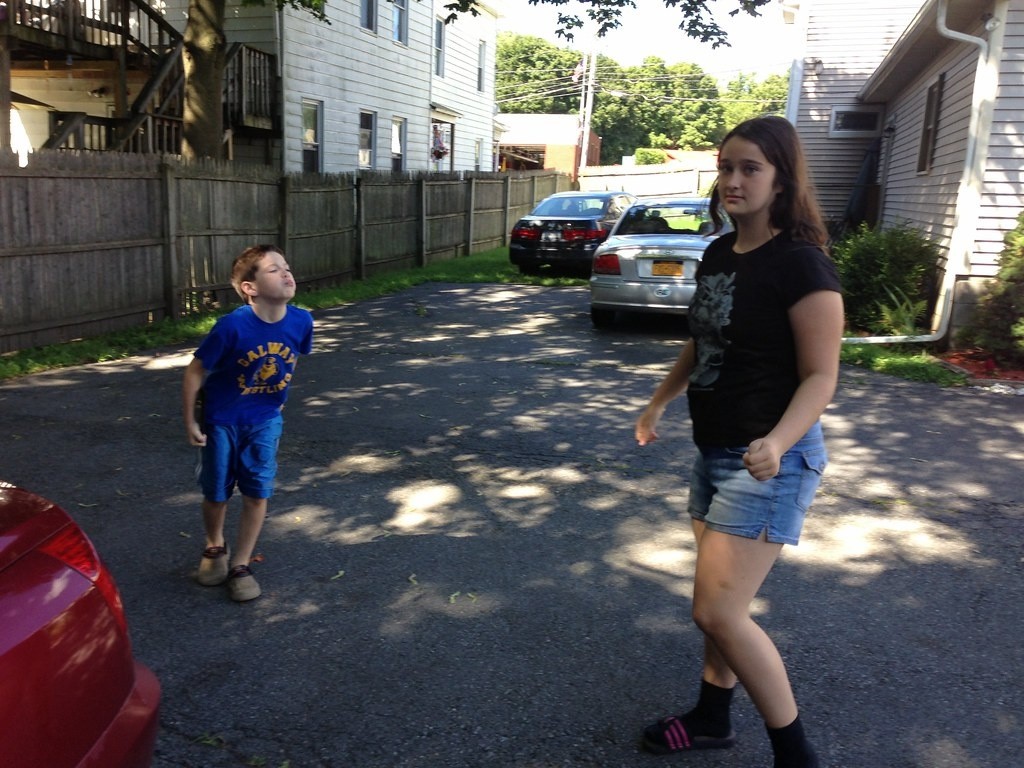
[508,190,639,276]
[589,198,736,330]
[0,482,162,767]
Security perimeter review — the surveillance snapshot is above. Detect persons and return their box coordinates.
[635,115,845,768]
[648,216,671,230]
[633,209,644,221]
[183,243,313,603]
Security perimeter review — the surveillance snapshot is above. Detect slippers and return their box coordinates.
[640,714,736,748]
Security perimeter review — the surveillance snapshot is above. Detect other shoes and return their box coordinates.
[226,566,260,602]
[198,541,230,587]
